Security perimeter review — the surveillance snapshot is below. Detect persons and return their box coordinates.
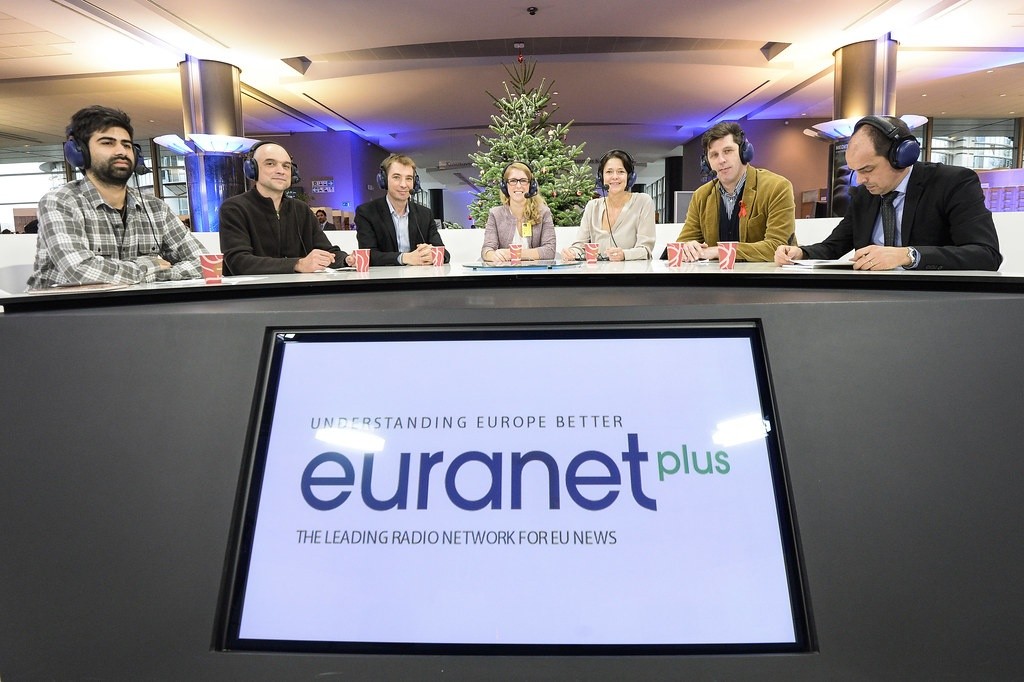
[773,115,1004,272]
[24,105,210,290]
[219,141,353,276]
[561,149,656,261]
[481,161,556,263]
[676,121,798,263]
[353,154,450,265]
[315,210,336,230]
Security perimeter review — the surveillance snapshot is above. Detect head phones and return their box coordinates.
[377,155,420,191]
[243,140,298,181]
[500,161,537,198]
[595,148,637,186]
[64,128,144,170]
[700,130,754,174]
[853,115,921,169]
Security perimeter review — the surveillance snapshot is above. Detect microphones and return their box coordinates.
[846,170,859,195]
[409,188,423,195]
[136,165,151,175]
[702,170,717,177]
[599,184,609,190]
[524,191,537,199]
[291,173,301,184]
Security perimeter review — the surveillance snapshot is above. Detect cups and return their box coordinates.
[667,243,684,267]
[717,242,739,269]
[431,246,444,266]
[200,254,224,284]
[509,244,522,265]
[355,249,371,272]
[584,243,599,263]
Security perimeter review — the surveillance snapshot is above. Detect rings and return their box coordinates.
[868,261,874,266]
[614,253,617,256]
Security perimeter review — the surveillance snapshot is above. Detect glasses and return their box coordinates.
[507,178,531,186]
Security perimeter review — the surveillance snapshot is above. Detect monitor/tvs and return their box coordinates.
[219,326,815,651]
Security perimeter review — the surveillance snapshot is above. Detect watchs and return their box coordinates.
[907,247,917,267]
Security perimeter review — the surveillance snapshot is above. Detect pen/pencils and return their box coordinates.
[50,280,112,288]
[782,264,813,269]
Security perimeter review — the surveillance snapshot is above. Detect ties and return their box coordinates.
[881,191,899,247]
